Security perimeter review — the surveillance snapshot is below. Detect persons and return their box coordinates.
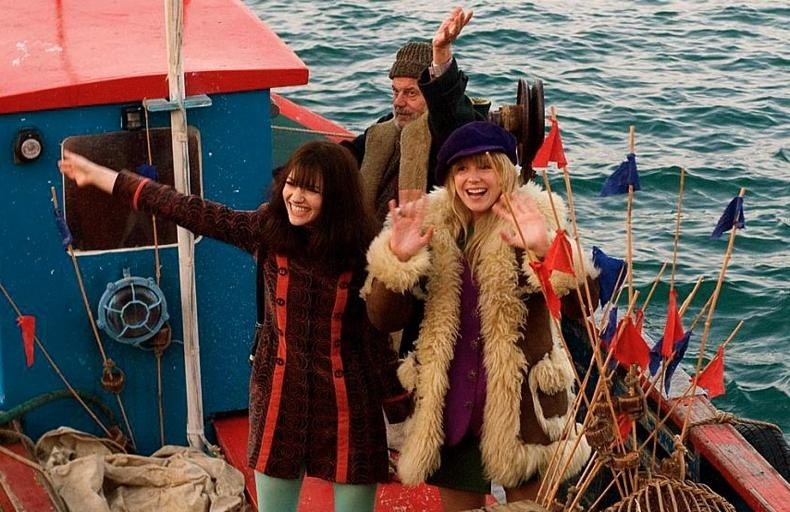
[58,142,411,511]
[340,5,492,225]
[369,121,599,494]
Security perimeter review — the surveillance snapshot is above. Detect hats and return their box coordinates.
[387,41,436,80]
[440,121,517,168]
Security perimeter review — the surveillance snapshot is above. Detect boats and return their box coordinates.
[0,0,790,512]
